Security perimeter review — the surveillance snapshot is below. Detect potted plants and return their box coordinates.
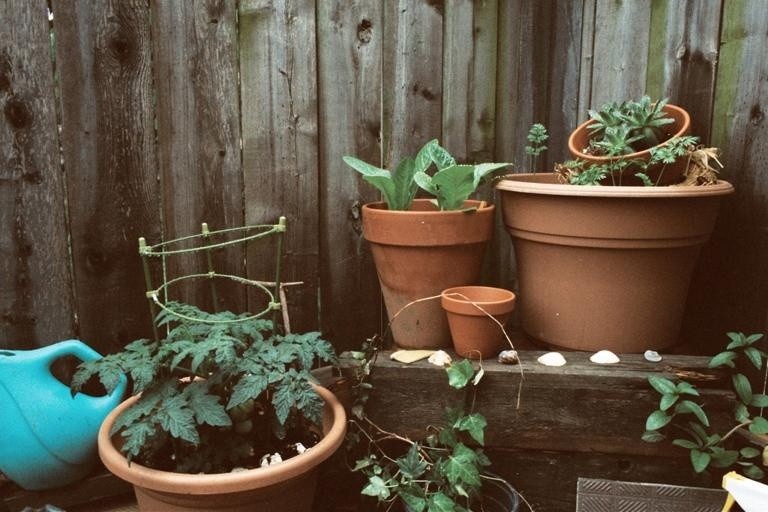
[341,138,515,349]
[566,93,694,188]
[63,299,348,510]
[494,122,734,354]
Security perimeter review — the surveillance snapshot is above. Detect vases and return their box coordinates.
[439,285,515,361]
